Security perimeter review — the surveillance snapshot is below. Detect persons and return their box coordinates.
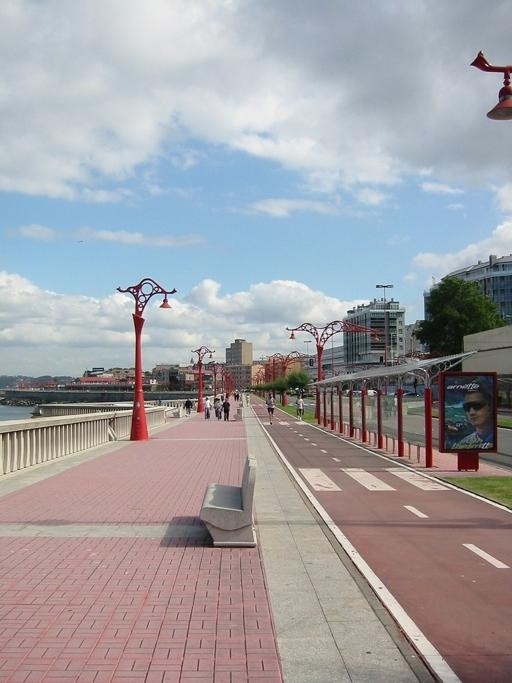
[294,395,306,419]
[455,386,494,449]
[183,398,192,418]
[203,390,240,421]
[265,394,276,425]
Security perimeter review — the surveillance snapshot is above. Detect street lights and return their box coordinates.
[190,346,236,413]
[303,340,312,374]
[117,277,179,440]
[374,283,394,368]
[470,49,512,122]
[254,349,311,406]
[285,320,382,424]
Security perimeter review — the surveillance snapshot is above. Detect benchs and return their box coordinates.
[199,454,258,547]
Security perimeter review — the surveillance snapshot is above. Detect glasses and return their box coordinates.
[462,400,488,411]
